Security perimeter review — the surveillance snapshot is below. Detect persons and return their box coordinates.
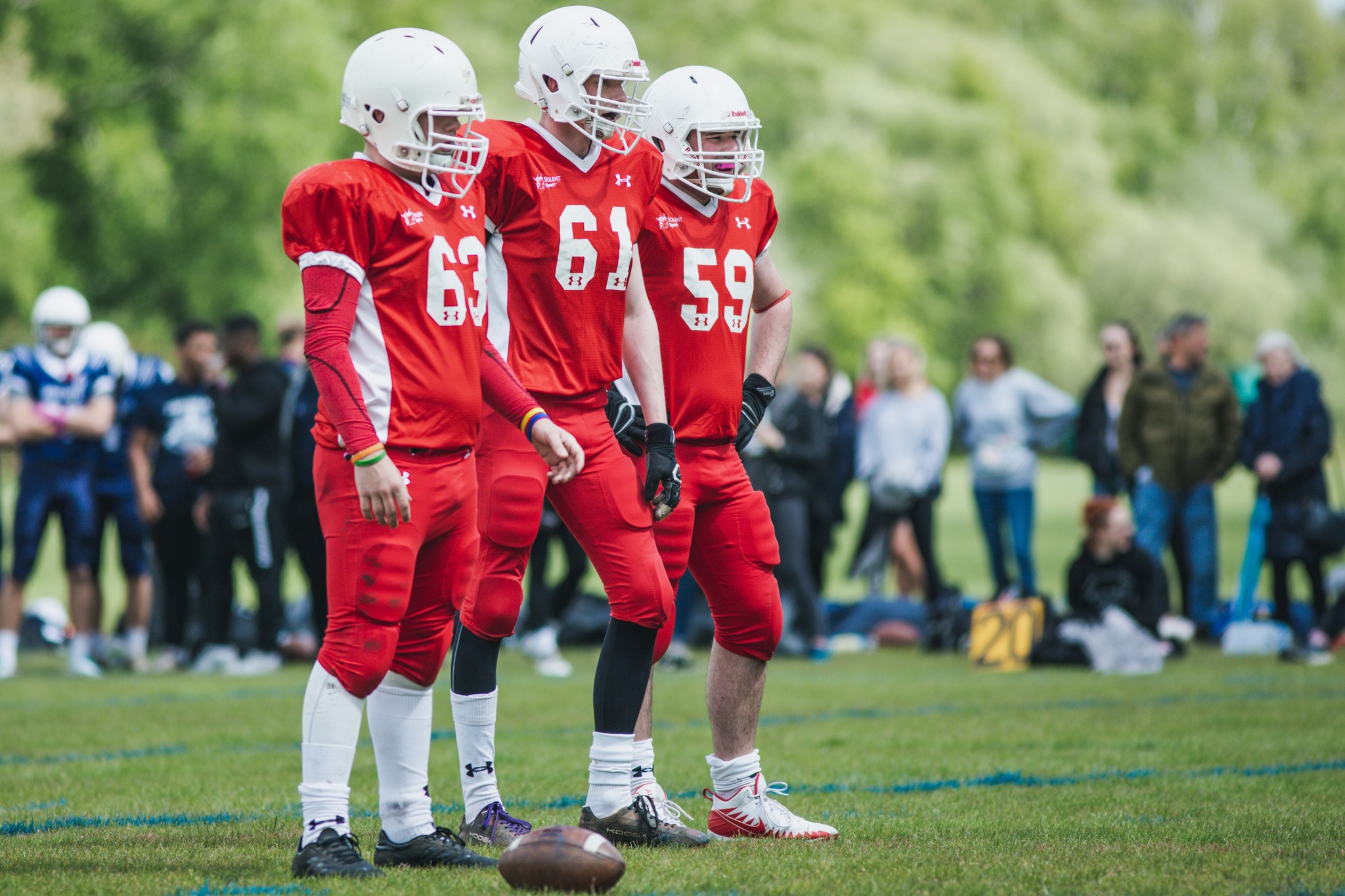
[609,66,837,840]
[0,285,1345,686]
[439,4,713,850]
[282,29,583,880]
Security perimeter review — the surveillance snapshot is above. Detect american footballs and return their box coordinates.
[498,825,627,894]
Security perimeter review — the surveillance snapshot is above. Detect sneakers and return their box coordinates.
[457,800,534,847]
[578,794,710,848]
[292,828,387,879]
[632,781,695,826]
[702,773,842,843]
[375,825,500,869]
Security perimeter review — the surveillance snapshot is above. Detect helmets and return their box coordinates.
[641,64,765,204]
[339,26,490,199]
[31,285,92,328]
[513,4,653,155]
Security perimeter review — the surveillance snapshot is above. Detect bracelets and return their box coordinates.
[527,413,550,443]
[520,407,545,433]
[354,449,387,466]
[351,442,385,464]
